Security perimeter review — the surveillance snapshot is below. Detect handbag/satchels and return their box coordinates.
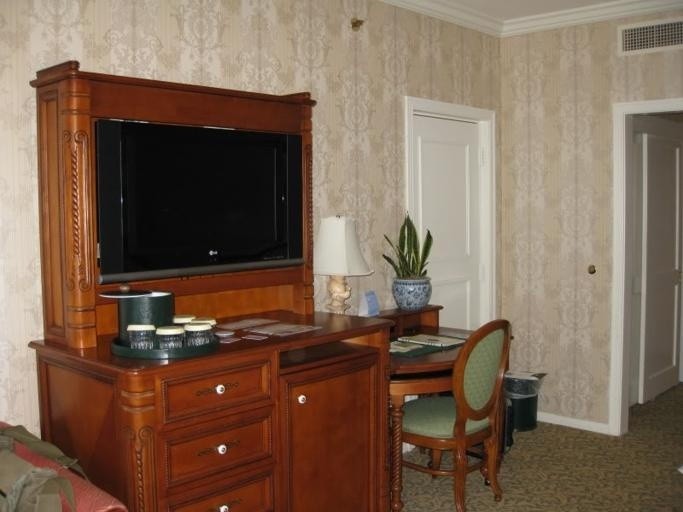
[0,448,61,512]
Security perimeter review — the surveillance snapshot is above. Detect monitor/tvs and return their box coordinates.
[93,118,304,285]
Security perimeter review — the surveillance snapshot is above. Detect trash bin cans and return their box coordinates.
[504,374,542,432]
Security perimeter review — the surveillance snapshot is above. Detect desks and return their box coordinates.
[390,324,474,509]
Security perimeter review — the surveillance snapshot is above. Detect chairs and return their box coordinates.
[401,317,513,509]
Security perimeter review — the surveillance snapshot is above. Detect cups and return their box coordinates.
[156,315,217,350]
[126,324,156,350]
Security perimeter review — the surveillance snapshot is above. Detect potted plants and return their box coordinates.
[382,215,439,308]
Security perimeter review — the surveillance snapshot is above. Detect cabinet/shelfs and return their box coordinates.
[25,312,405,509]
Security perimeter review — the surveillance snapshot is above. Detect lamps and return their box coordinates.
[310,217,375,314]
